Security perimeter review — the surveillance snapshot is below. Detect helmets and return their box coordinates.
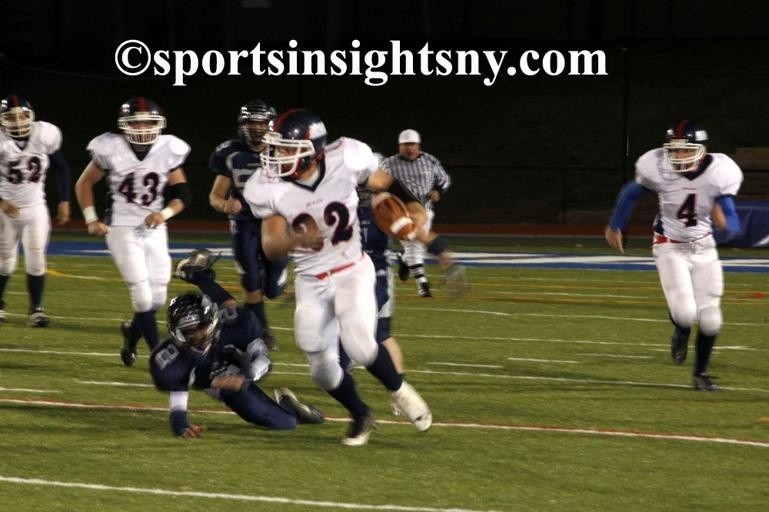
[664,117,711,174]
[0,95,35,140]
[167,291,220,352]
[237,99,278,155]
[260,107,330,182]
[117,95,167,146]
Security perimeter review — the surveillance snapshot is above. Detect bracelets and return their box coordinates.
[427,234,449,255]
[170,409,189,434]
[158,206,175,221]
[82,205,99,225]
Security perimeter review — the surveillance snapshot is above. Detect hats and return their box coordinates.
[398,129,422,144]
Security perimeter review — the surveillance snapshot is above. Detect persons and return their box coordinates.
[242,107,433,447]
[0,93,70,327]
[209,99,289,351]
[339,188,464,416]
[150,250,325,439]
[378,128,451,298]
[74,97,191,367]
[605,120,744,392]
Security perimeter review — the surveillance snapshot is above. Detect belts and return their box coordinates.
[653,232,712,244]
[316,257,363,280]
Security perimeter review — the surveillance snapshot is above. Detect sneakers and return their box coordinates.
[692,368,718,393]
[399,256,410,281]
[119,320,138,368]
[26,304,51,328]
[391,378,433,432]
[341,408,375,449]
[669,333,689,366]
[416,276,432,297]
[273,385,325,424]
[176,249,215,286]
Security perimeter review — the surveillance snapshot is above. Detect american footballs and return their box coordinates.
[370,191,414,241]
